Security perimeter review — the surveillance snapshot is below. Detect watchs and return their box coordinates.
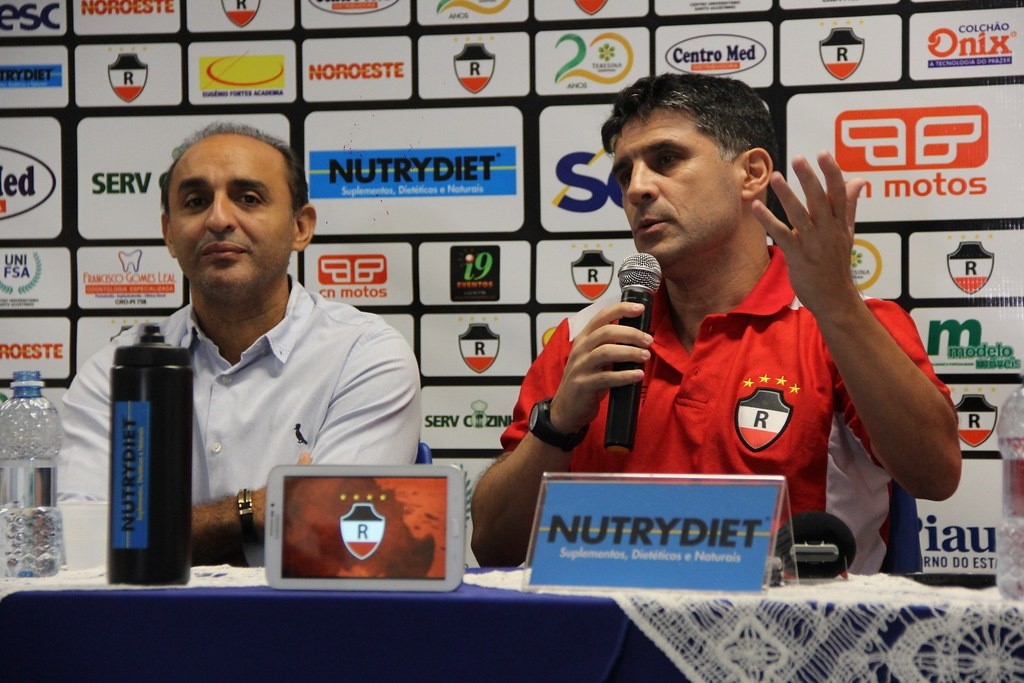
[528,398,590,449]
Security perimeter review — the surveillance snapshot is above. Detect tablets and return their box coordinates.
[264,464,466,592]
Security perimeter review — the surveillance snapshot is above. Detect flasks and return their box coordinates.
[108,325,194,587]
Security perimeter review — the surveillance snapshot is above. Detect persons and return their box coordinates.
[470,71,962,568]
[55,123,422,572]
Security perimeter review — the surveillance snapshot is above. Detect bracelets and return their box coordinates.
[237,489,259,545]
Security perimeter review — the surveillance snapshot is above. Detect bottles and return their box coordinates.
[996,387,1024,601]
[0,372,63,578]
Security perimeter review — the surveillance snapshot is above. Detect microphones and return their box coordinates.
[604,253,663,454]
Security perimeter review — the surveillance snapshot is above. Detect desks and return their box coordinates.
[0,568,1024,683]
